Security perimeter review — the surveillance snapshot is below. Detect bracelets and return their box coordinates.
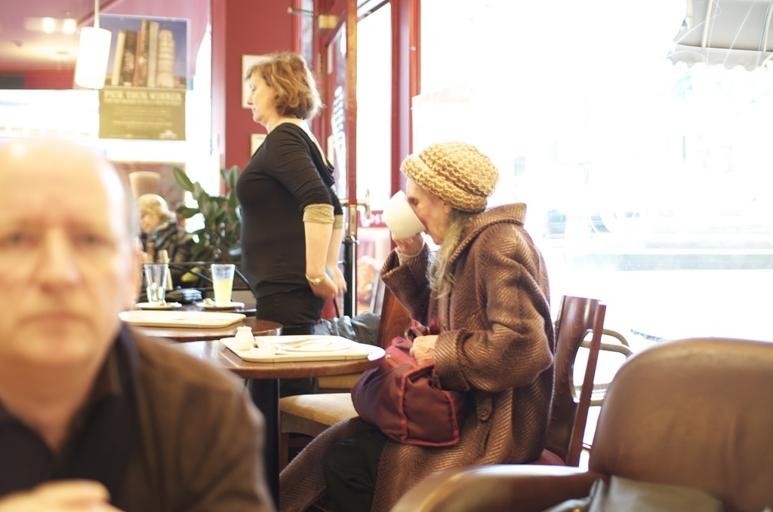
[305,271,327,288]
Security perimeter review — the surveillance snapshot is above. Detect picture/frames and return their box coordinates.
[249,132,267,158]
[240,53,272,109]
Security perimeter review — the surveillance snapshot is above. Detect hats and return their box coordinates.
[400,142,500,213]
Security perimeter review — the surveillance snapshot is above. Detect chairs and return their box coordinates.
[390,340,773,512]
[535,295,606,466]
[279,272,411,472]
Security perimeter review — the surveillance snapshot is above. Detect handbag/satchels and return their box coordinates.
[351,318,462,447]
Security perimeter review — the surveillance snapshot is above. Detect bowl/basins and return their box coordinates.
[383,189,426,242]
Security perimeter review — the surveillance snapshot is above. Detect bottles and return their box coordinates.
[234,326,256,353]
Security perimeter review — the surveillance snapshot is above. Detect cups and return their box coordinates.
[143,263,169,305]
[210,262,236,305]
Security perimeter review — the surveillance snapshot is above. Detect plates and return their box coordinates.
[198,301,246,310]
[135,302,183,311]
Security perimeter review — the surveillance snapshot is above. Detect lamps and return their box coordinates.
[74,0,112,89]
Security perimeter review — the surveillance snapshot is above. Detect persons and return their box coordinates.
[136,189,196,282]
[0,110,278,511]
[236,54,349,511]
[277,144,557,511]
